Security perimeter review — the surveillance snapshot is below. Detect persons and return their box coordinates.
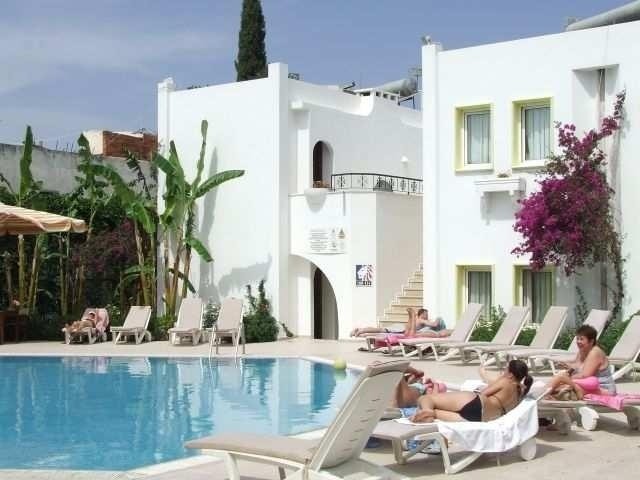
[545,325,617,401]
[65,308,98,333]
[350,307,455,337]
[390,360,533,423]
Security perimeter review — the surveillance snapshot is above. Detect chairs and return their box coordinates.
[182,357,417,480]
[357,304,640,389]
[109,305,153,346]
[374,357,640,475]
[166,295,207,347]
[210,296,248,356]
[61,307,109,346]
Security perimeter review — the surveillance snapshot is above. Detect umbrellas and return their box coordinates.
[1,202,88,235]
